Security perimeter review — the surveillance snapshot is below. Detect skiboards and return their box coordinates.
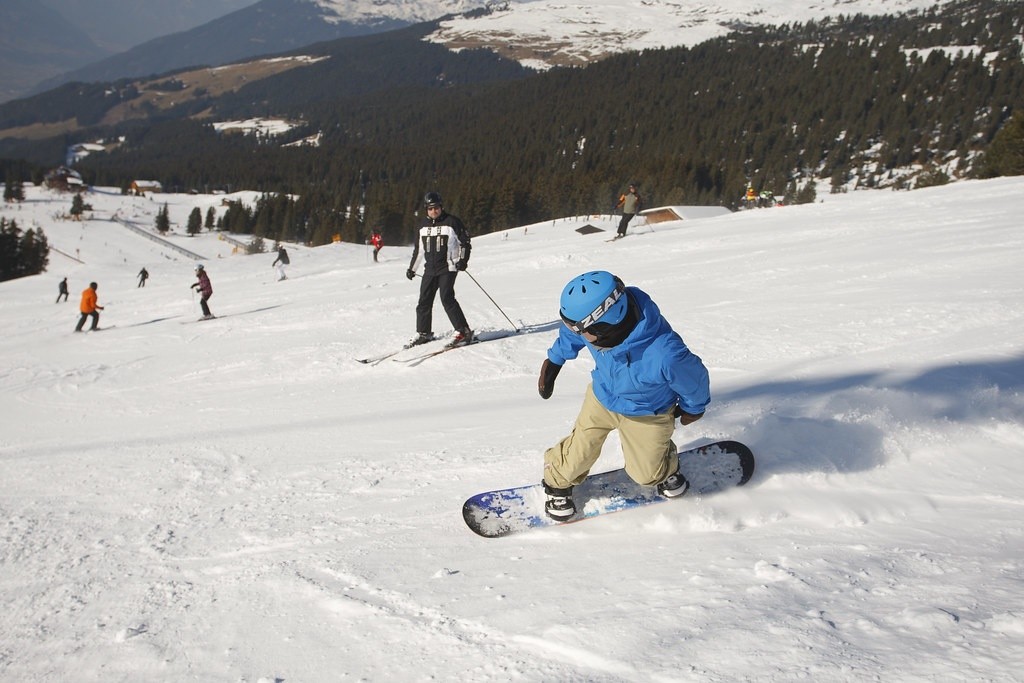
[604,233,635,243]
[353,330,509,368]
[82,324,115,335]
[180,314,227,325]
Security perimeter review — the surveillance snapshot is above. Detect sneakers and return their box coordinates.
[658,470,687,499]
[403,332,431,349]
[542,479,575,521]
[446,330,472,348]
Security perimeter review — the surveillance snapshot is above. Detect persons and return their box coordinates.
[612,184,642,240]
[136,267,149,288]
[742,187,785,209]
[272,244,290,281]
[367,229,384,261]
[74,282,105,332]
[190,265,213,321]
[538,271,712,522]
[403,192,478,349]
[56,277,69,303]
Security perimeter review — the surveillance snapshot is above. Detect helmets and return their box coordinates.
[194,264,204,273]
[560,271,627,325]
[425,192,444,215]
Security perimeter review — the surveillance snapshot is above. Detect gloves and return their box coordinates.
[406,269,415,280]
[455,261,467,271]
[538,358,562,399]
[674,404,705,426]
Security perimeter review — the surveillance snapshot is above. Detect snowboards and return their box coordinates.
[462,441,757,539]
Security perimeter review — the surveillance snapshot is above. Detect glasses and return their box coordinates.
[559,312,581,337]
[427,205,438,211]
